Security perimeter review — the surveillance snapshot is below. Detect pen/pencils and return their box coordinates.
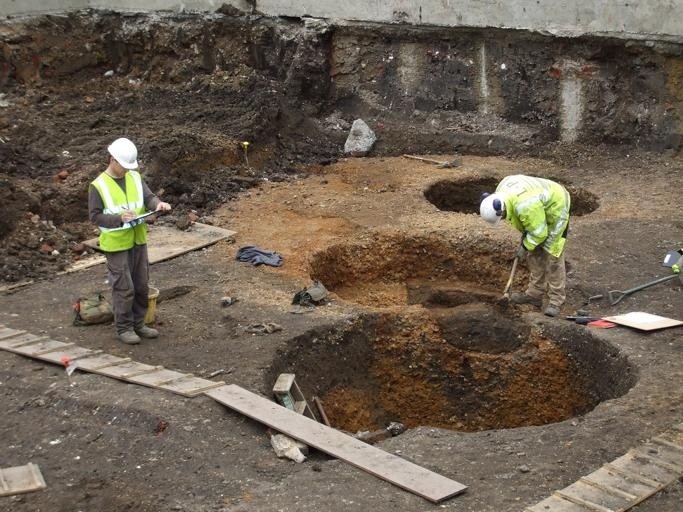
[121,206,127,211]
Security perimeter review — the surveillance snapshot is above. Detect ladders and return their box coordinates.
[272,373,318,422]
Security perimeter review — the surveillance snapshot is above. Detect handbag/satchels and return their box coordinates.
[292,279,327,308]
[74,292,114,327]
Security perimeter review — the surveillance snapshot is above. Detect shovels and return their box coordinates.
[608,255,682,306]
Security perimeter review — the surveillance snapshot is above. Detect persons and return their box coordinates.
[480,175,574,316]
[88,137,173,342]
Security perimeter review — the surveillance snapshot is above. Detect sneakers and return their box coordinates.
[545,303,561,316]
[511,291,542,306]
[115,324,158,346]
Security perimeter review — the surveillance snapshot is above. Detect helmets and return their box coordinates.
[107,137,140,170]
[481,192,505,227]
